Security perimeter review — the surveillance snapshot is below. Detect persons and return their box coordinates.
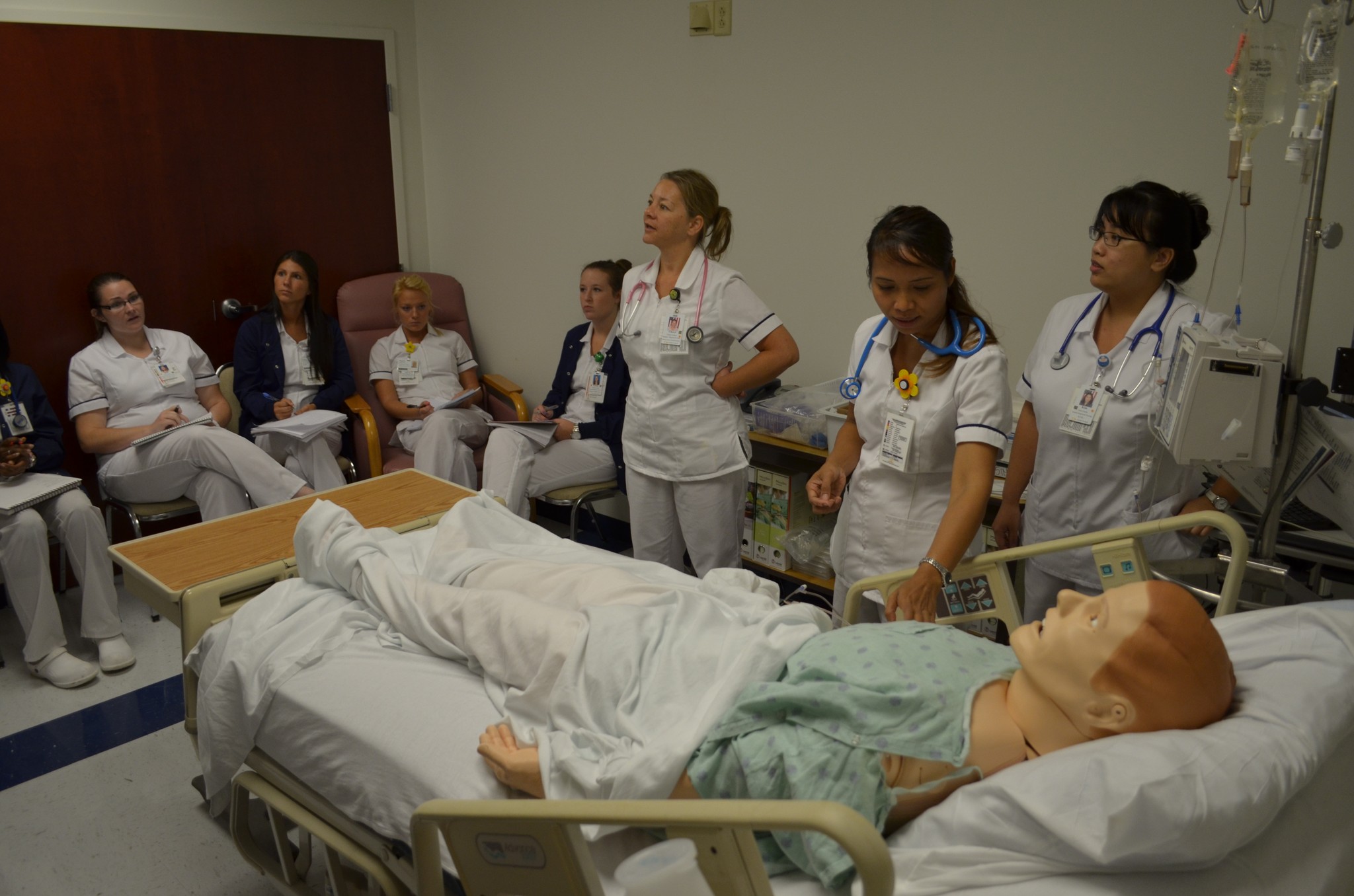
[990,181,1261,626]
[67,271,315,522]
[233,249,356,494]
[291,490,1237,840]
[804,204,1014,630]
[618,168,800,581]
[482,258,633,521]
[369,273,495,490]
[0,323,136,689]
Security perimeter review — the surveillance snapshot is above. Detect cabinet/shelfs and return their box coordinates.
[737,431,842,591]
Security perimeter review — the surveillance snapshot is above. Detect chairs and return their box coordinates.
[101,493,201,619]
[214,365,358,484]
[104,468,1354,896]
[535,474,629,548]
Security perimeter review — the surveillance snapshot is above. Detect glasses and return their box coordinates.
[1088,226,1153,247]
[97,292,143,312]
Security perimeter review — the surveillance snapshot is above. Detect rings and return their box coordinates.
[8,450,11,455]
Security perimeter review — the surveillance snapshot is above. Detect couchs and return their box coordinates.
[336,270,536,524]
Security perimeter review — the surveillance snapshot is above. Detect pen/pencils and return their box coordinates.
[4,441,24,445]
[533,404,559,414]
[407,404,420,408]
[262,392,280,402]
[174,404,179,412]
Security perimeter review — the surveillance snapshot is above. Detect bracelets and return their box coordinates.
[24,453,36,469]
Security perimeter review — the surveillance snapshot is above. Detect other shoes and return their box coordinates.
[92,632,136,671]
[26,647,99,688]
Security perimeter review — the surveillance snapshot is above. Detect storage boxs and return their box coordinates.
[748,387,849,450]
[817,399,853,454]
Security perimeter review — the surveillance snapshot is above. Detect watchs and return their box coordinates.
[570,423,581,440]
[919,557,952,589]
[1206,488,1231,511]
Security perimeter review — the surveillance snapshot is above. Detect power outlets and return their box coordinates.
[713,0,731,35]
[688,1,714,36]
